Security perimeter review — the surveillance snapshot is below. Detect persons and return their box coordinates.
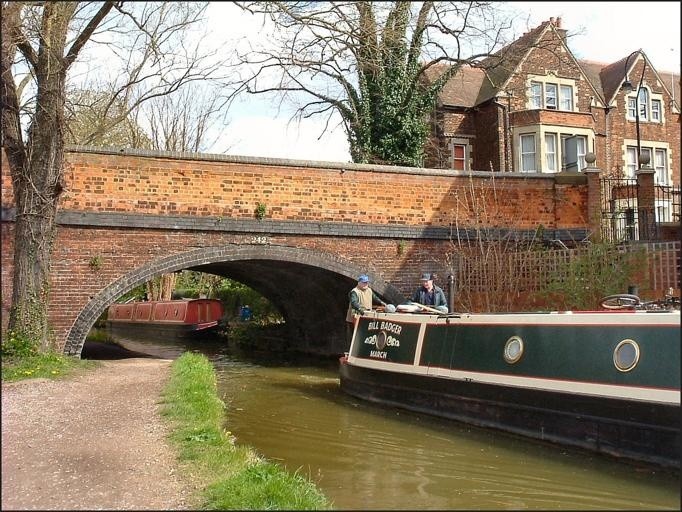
[344,275,386,352]
[407,273,448,313]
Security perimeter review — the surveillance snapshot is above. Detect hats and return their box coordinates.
[359,275,369,282]
[419,274,432,280]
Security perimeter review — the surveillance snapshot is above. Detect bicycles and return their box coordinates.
[597,286,680,312]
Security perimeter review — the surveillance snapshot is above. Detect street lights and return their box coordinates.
[622,51,647,172]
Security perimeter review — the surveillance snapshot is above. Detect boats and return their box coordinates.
[103,296,224,343]
[337,305,681,478]
